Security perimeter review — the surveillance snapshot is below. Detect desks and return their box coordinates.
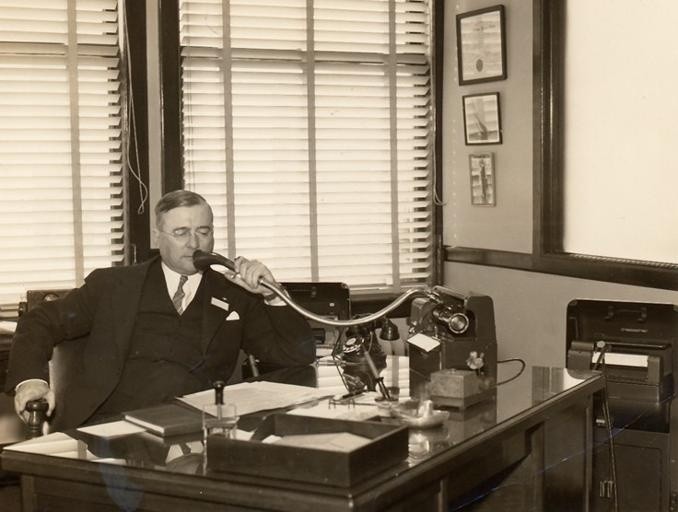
[0,356,606,512]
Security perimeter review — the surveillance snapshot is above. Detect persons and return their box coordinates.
[479,160,489,200]
[3,189,317,435]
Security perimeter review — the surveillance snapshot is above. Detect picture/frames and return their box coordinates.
[455,5,508,86]
[468,153,496,207]
[463,91,502,145]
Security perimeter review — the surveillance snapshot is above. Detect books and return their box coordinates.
[123,404,215,438]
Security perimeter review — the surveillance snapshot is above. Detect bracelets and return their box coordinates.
[265,283,286,303]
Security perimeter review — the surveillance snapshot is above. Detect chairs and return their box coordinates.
[18,290,78,439]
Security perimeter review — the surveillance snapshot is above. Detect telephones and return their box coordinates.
[332,313,399,370]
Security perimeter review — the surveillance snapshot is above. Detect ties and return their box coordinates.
[171,274,188,316]
